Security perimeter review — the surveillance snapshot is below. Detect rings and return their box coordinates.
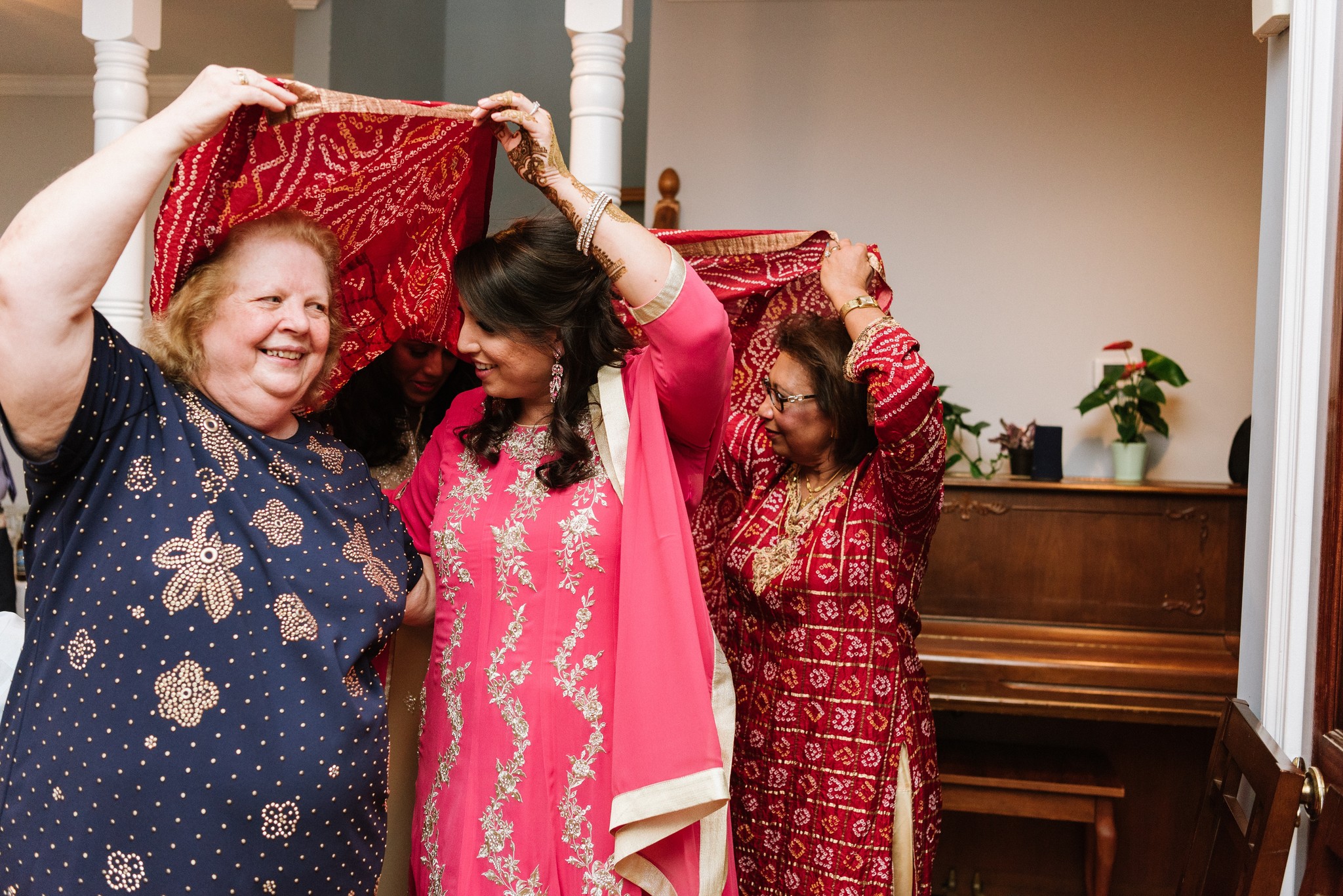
[829,246,840,253]
[820,249,831,258]
[526,100,542,116]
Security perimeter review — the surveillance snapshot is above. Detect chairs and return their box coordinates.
[1180,695,1304,895]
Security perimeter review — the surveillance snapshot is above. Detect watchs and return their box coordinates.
[838,296,883,325]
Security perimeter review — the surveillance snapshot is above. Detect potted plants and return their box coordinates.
[987,418,1038,476]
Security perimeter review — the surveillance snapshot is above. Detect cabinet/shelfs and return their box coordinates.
[914,470,1250,639]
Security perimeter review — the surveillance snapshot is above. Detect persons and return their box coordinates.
[0,63,441,894]
[344,88,739,895]
[311,323,483,892]
[716,240,954,896]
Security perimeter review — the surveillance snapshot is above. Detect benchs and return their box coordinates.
[913,612,1236,804]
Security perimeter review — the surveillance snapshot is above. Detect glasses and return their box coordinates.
[761,377,818,413]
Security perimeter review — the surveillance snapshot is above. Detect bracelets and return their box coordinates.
[574,191,612,258]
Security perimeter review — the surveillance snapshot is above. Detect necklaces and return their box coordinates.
[800,463,850,510]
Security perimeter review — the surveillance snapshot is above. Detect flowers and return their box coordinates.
[1071,341,1190,445]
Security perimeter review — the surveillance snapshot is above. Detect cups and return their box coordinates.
[1030,425,1062,483]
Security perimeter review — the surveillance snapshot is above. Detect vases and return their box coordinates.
[1110,439,1150,486]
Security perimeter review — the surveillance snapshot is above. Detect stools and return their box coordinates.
[935,762,1124,895]
